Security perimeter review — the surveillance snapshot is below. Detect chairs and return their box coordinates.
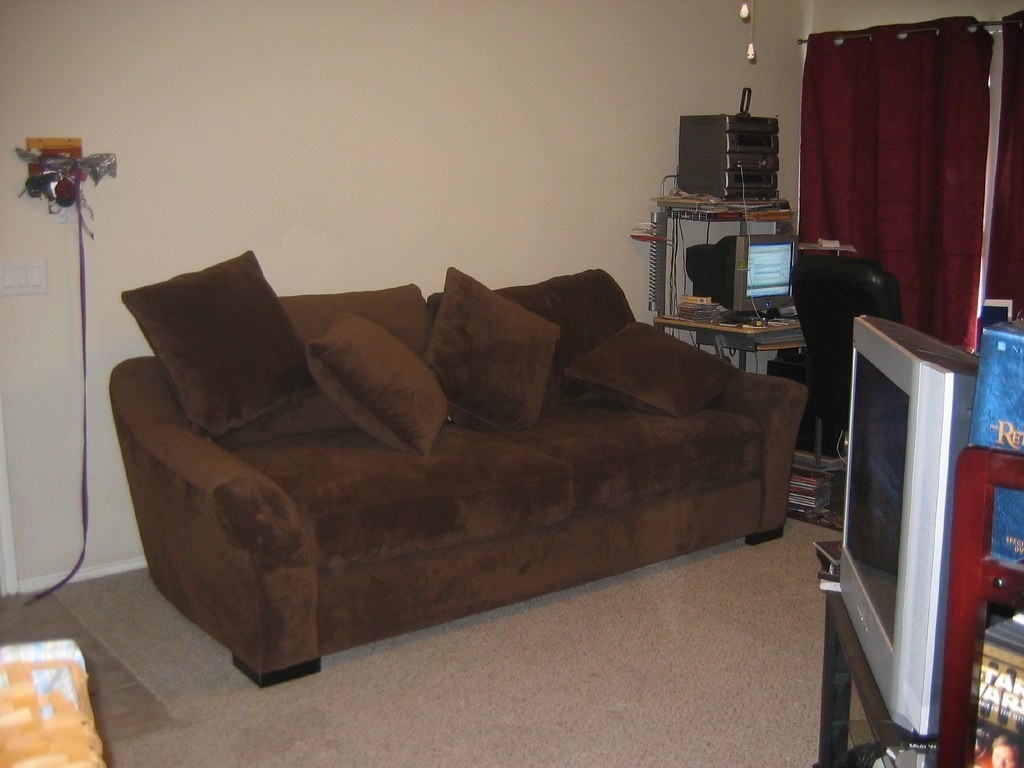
[766,254,904,475]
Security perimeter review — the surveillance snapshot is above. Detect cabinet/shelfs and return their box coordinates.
[812,541,939,768]
[655,197,806,374]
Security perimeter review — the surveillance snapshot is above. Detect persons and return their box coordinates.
[991,732,1022,768]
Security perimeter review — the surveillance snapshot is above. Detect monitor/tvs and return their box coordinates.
[838,314,981,737]
[686,235,799,313]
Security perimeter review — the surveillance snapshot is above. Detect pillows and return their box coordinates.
[307,312,449,459]
[119,250,317,442]
[565,321,733,418]
[423,266,562,432]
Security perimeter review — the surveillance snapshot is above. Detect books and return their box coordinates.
[972,614,1024,768]
[787,451,847,509]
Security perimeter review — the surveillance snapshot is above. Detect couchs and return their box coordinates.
[107,271,810,689]
